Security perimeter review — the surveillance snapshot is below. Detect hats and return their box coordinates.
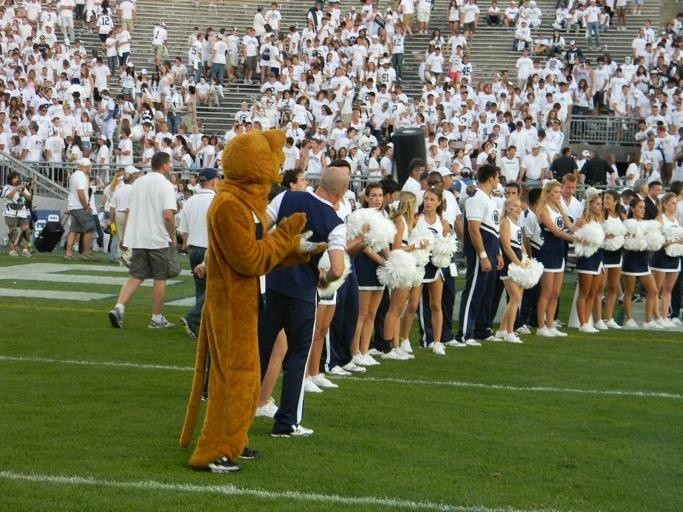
[81,157,92,166]
[28,124,38,130]
[97,135,107,142]
[582,150,589,157]
[198,167,221,182]
[463,144,473,153]
[434,101,467,126]
[532,143,540,148]
[643,159,650,165]
[584,187,602,198]
[124,165,141,174]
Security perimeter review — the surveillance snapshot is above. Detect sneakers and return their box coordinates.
[418,318,567,355]
[578,315,682,333]
[270,424,315,438]
[303,338,415,393]
[108,307,127,328]
[617,292,641,306]
[255,396,278,418]
[9,249,32,257]
[148,315,177,329]
[179,315,198,340]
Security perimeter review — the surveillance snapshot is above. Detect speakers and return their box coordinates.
[392,127,427,186]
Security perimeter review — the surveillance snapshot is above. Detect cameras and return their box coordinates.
[16,178,32,190]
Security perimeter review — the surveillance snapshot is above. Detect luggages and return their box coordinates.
[34,211,71,252]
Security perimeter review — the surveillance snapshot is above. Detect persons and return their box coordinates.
[0,0,683,269]
[104,127,683,474]
